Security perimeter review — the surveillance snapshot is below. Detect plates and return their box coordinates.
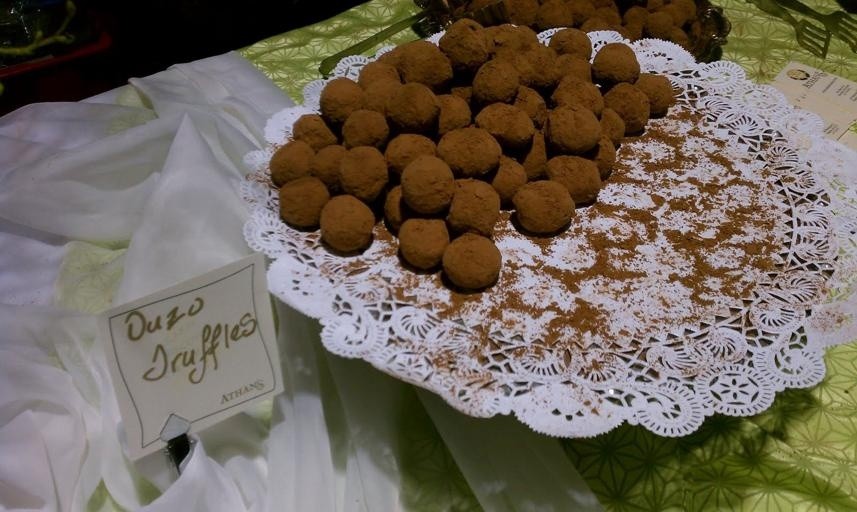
[415,1,732,64]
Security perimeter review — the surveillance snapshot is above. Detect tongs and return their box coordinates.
[747,0,857,58]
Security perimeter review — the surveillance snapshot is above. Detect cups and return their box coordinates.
[0,1,34,48]
